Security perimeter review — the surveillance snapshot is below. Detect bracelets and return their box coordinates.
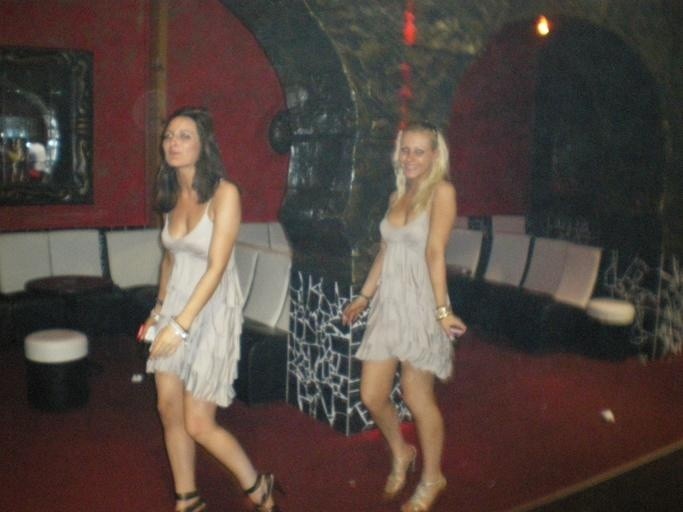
[150,309,159,321]
[154,298,163,306]
[436,306,453,320]
[359,293,371,302]
[168,317,189,341]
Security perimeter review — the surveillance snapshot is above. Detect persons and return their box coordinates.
[137,105,287,512]
[340,121,467,512]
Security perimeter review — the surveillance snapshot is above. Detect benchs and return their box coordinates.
[0,223,291,405]
[444,215,602,352]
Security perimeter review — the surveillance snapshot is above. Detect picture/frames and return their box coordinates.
[0,45,95,206]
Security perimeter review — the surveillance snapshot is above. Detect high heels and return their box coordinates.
[385,445,416,494]
[400,478,447,512]
[242,474,285,512]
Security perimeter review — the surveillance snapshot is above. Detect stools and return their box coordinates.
[583,296,635,359]
